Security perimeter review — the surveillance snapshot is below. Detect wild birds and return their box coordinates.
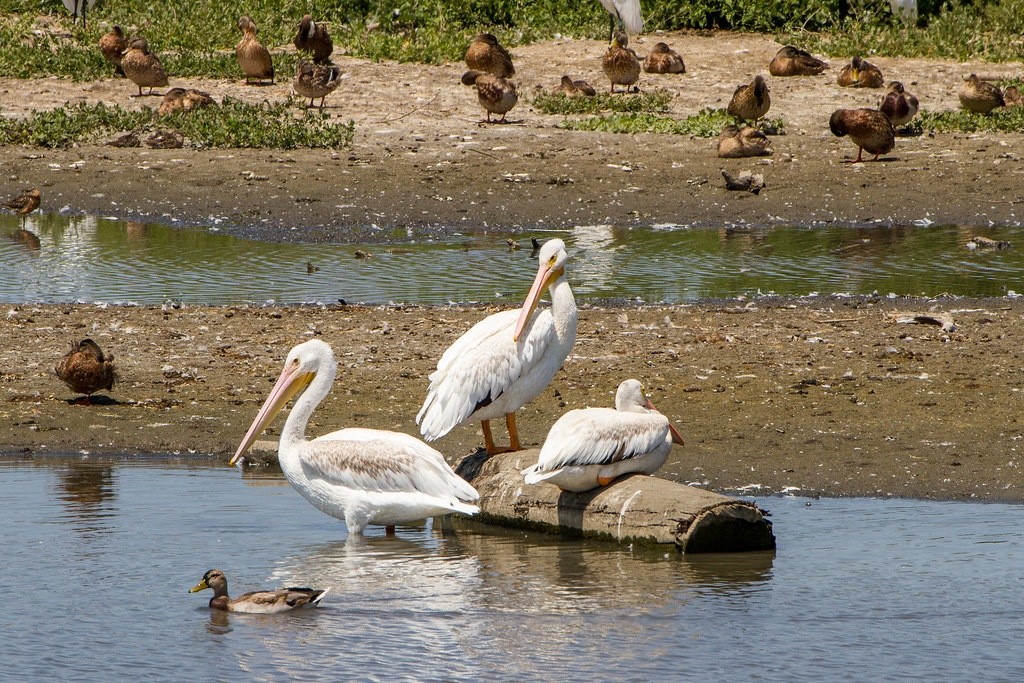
[62,0,95,28]
[236,16,273,84]
[718,46,1004,190]
[121,36,169,97]
[159,87,218,116]
[293,60,344,108]
[971,236,1005,249]
[98,21,126,74]
[465,34,516,80]
[462,70,519,122]
[106,130,184,148]
[3,187,41,225]
[525,379,685,498]
[55,339,115,401]
[229,339,482,540]
[415,239,579,457]
[294,16,333,61]
[188,569,330,614]
[600,0,643,44]
[560,28,684,97]
